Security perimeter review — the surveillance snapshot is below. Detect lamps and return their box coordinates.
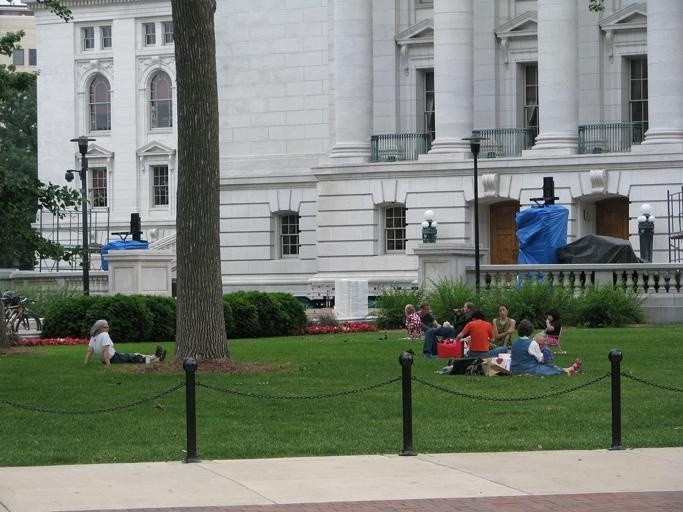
[637,203,656,226]
[420,209,438,229]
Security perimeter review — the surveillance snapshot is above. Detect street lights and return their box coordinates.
[462,130,490,302]
[65,136,96,296]
[637,204,656,263]
[421,209,437,242]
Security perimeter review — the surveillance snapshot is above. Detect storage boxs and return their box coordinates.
[438,340,464,358]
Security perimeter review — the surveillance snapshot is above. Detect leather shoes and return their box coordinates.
[155,346,162,358]
[571,358,582,372]
[158,350,167,361]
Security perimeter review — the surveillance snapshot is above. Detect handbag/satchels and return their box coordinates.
[466,356,486,377]
[437,338,465,357]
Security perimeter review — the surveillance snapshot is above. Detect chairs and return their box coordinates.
[545,325,563,352]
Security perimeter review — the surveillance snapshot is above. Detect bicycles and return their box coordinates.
[1,290,41,334]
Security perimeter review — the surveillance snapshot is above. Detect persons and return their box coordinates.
[84,319,166,366]
[405,302,581,377]
